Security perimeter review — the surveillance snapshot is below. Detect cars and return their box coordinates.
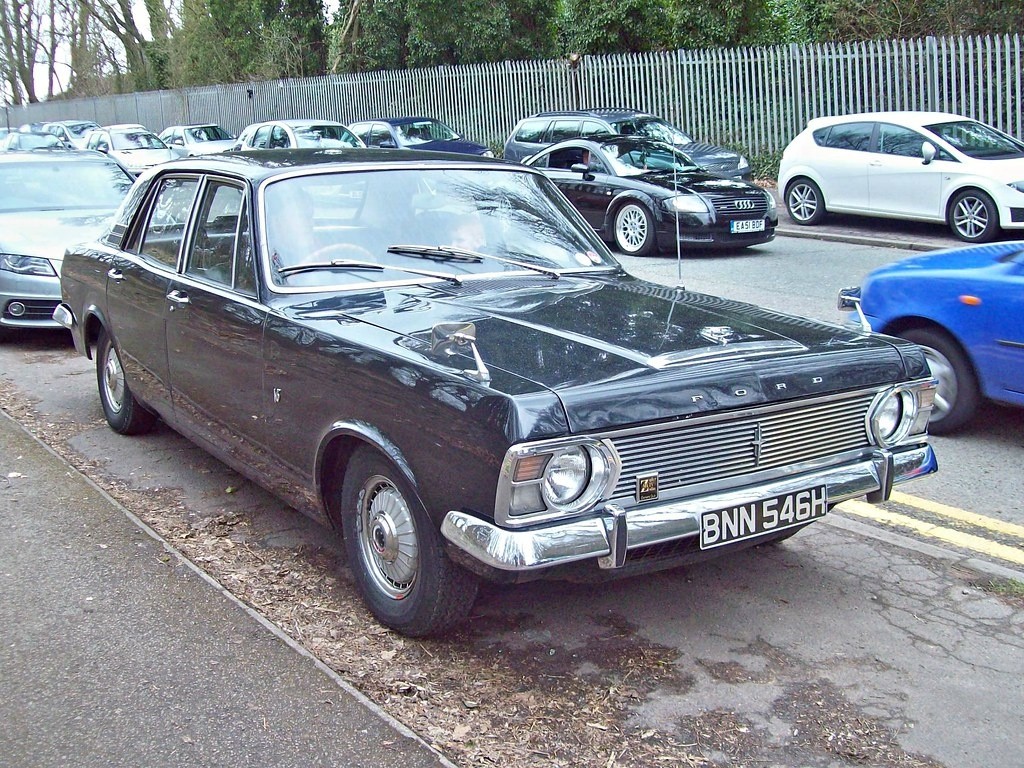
[53,146,939,641]
[839,241,1024,436]
[229,119,367,152]
[778,110,1024,243]
[0,122,51,144]
[0,132,69,153]
[77,123,172,181]
[518,133,778,257]
[339,116,495,160]
[0,149,142,327]
[159,122,240,160]
[42,119,102,151]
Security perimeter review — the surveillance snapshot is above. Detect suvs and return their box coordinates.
[505,108,755,185]
